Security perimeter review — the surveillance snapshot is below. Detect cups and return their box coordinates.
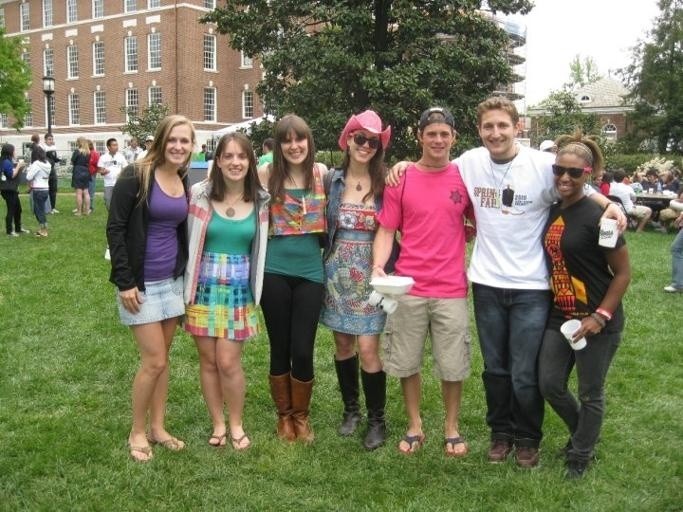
[368,290,399,315]
[560,318,588,352]
[597,218,622,249]
[641,187,654,195]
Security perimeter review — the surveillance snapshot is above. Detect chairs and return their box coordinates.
[604,193,637,232]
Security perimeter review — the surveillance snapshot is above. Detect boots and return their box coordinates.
[360,366,387,451]
[332,352,362,437]
[268,370,316,444]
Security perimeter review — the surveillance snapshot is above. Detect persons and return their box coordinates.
[384,96,629,469]
[198,144,210,163]
[257,137,274,167]
[325,109,400,452]
[255,112,328,445]
[370,106,476,456]
[105,114,196,461]
[537,128,632,474]
[0,133,155,238]
[182,131,271,451]
[590,167,683,293]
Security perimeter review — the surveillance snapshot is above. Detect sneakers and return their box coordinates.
[663,285,683,294]
[559,435,600,484]
[7,228,29,237]
[49,207,96,217]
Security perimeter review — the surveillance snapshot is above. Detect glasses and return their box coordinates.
[552,163,592,179]
[349,133,381,149]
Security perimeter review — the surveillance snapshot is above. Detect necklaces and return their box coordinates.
[349,165,367,192]
[418,162,449,169]
[490,145,517,193]
[226,191,245,217]
[157,167,180,196]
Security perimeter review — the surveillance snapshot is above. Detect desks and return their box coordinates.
[630,193,674,227]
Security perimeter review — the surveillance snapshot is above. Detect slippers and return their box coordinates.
[33,231,49,238]
[398,432,426,455]
[147,434,187,452]
[208,433,228,448]
[229,429,251,451]
[128,439,154,465]
[444,434,468,458]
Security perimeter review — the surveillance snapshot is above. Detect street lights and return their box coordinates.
[41,70,54,134]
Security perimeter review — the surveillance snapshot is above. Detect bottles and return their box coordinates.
[636,183,640,196]
[656,179,662,194]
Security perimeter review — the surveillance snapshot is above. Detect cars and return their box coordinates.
[55,152,74,175]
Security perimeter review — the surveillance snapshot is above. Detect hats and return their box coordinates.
[419,107,455,129]
[338,110,392,154]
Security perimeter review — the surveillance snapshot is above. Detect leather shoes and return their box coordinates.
[487,438,513,463]
[515,445,539,469]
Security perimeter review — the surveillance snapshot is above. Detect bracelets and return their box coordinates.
[372,264,384,270]
[595,307,612,321]
[591,313,606,328]
